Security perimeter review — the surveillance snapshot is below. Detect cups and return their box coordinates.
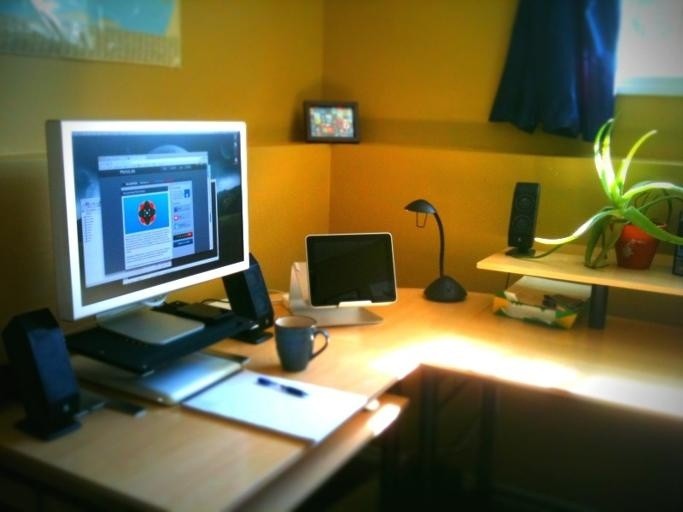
[274,314,330,374]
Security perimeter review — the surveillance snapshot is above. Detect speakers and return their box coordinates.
[508,182,539,249]
[0,308,81,440]
[223,254,274,339]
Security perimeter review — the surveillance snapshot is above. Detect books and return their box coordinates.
[179,367,369,444]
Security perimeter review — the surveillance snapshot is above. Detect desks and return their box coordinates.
[0,287,683,512]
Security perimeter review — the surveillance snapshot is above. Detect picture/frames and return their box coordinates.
[305,100,360,143]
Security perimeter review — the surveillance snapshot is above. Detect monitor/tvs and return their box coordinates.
[45,118,250,346]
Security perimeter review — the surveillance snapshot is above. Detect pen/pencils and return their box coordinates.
[258,378,308,398]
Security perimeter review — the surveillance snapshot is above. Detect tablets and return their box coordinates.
[304,231,398,309]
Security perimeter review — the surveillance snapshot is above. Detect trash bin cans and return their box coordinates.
[377,452,468,512]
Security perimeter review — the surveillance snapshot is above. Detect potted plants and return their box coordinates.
[531,111,683,273]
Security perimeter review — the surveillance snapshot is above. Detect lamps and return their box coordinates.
[406,199,467,304]
